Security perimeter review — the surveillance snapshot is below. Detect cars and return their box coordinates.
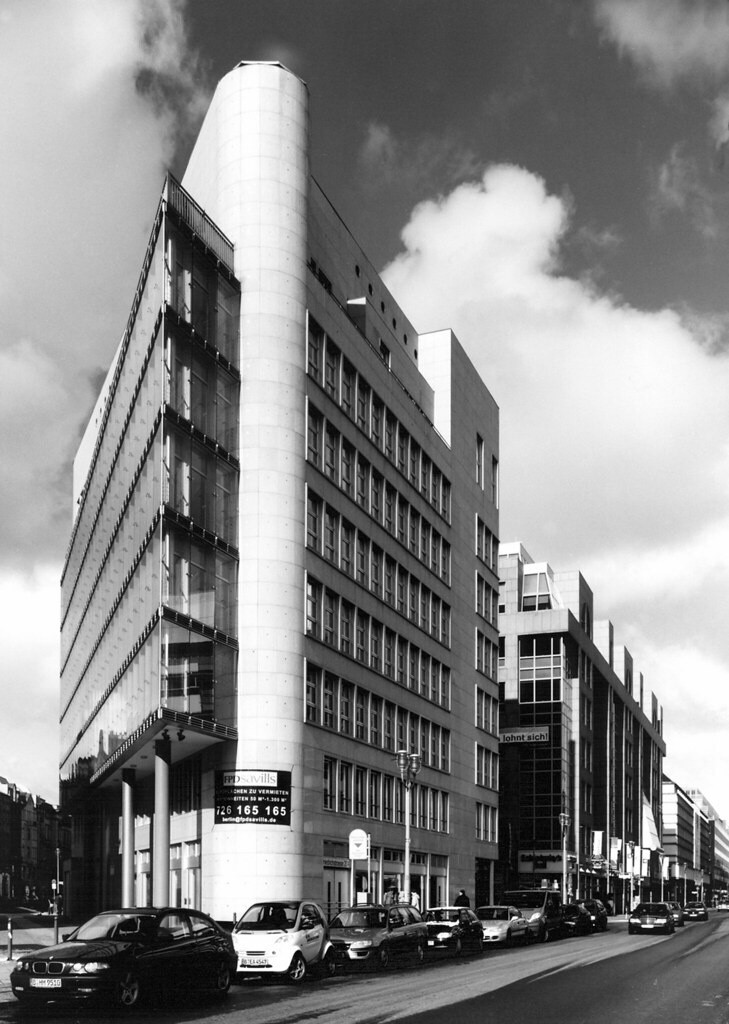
[620,898,729,936]
[10,885,610,1018]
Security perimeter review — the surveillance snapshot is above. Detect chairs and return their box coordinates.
[120,919,137,935]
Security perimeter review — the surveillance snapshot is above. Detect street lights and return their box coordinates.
[628,840,636,913]
[659,852,666,903]
[559,812,572,903]
[701,868,705,904]
[392,746,423,901]
[683,862,689,907]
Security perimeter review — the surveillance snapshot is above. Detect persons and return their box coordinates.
[410,889,420,912]
[454,889,470,909]
